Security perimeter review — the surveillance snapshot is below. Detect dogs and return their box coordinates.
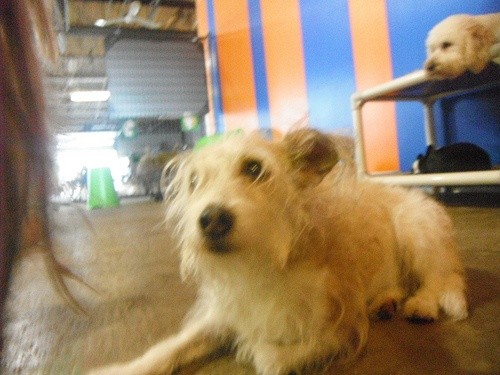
[88,125,468,375]
[423,12,500,80]
